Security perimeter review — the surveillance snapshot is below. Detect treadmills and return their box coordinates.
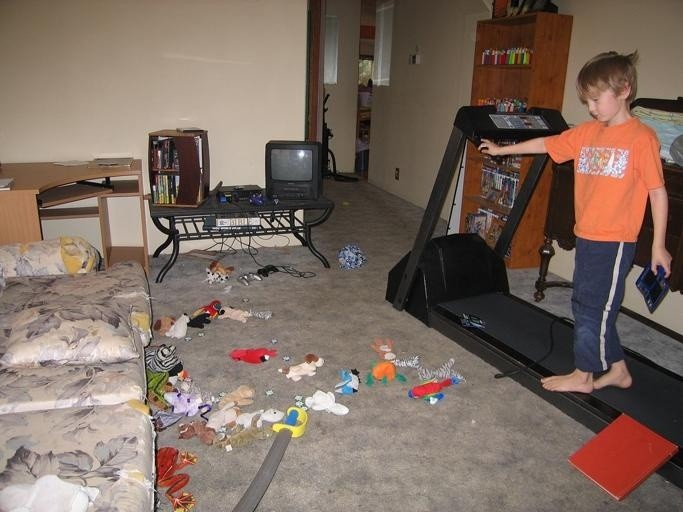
[384,105,682,488]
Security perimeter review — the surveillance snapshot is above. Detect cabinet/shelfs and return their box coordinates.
[458,11,575,269]
[147,128,210,208]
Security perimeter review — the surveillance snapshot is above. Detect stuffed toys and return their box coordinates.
[365,335,465,406]
[156,447,198,512]
[146,300,361,449]
[202,260,234,285]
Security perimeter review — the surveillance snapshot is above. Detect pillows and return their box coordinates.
[0,301,140,369]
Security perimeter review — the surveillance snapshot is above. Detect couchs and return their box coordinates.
[0,235,158,512]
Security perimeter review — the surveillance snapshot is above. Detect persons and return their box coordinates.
[478,48,673,396]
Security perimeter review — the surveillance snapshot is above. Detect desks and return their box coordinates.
[356,107,371,141]
[535,158,683,304]
[147,194,337,284]
[0,159,151,275]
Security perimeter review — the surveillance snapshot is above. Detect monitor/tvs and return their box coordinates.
[265,140,323,201]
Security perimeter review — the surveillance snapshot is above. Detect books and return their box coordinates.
[568,411,679,501]
[464,138,521,240]
[150,139,180,205]
